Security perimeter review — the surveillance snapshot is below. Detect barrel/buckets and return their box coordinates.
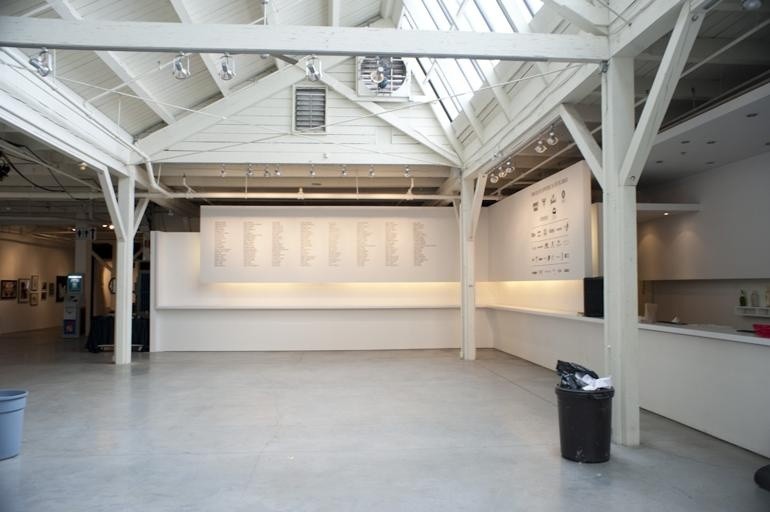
[0,389,29,460]
[555,385,614,463]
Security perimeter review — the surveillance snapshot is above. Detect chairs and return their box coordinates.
[637,301,770,339]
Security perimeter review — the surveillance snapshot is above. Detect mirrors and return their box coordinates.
[108,277,116,294]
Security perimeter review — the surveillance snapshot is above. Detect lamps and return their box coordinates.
[217,49,235,81]
[218,162,414,180]
[171,49,194,81]
[303,53,321,81]
[28,46,57,79]
[487,130,561,185]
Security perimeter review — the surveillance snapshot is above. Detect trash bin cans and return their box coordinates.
[0,389,29,461]
[555,386,615,465]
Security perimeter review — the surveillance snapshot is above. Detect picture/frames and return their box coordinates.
[0,273,69,309]
